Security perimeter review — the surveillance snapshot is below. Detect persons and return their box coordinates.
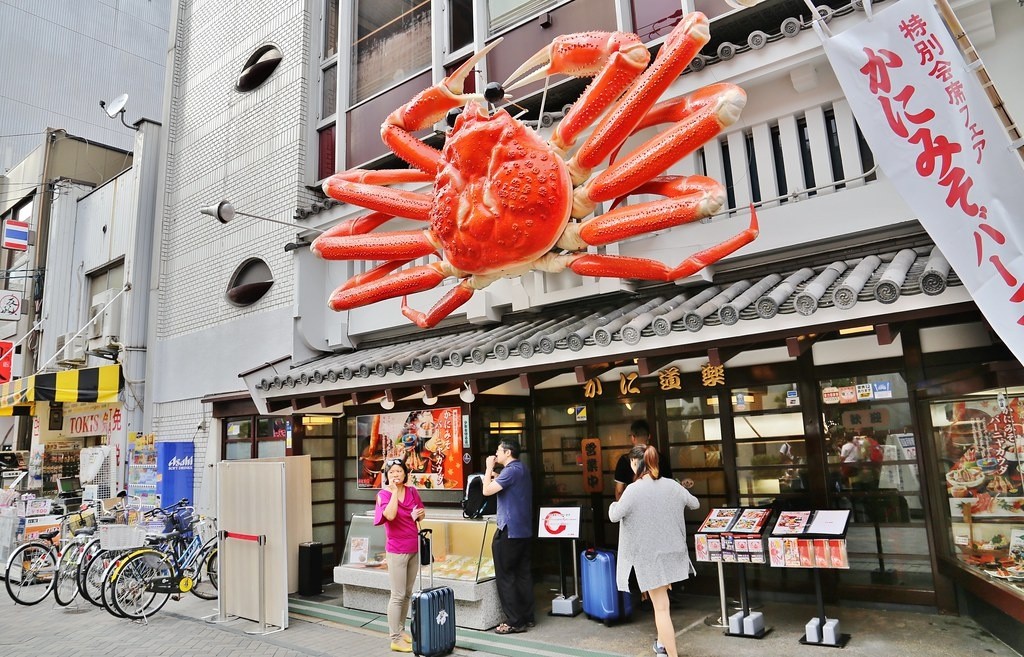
[614,420,679,611]
[841,434,884,479]
[608,444,700,657]
[373,458,425,653]
[483,438,536,634]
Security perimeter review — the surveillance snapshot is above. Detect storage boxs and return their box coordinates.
[100,524,147,551]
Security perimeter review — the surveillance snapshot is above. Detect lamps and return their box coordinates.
[380,396,395,410]
[421,387,438,405]
[459,384,475,403]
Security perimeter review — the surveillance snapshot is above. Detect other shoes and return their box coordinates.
[403,637,412,642]
[391,642,412,652]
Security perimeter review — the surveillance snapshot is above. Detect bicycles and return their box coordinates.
[5,489,219,626]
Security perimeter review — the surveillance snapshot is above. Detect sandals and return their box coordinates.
[526,622,534,626]
[495,620,527,633]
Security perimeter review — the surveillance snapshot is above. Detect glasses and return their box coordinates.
[387,460,402,466]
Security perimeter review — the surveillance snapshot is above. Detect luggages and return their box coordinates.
[409,528,456,657]
[580,546,631,627]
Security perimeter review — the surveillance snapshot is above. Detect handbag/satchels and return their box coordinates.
[420,535,434,566]
[460,474,490,520]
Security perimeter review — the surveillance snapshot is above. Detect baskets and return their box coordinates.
[138,521,164,533]
[99,523,147,551]
[67,512,97,537]
[196,517,217,549]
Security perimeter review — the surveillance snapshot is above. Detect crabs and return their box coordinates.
[308,10,759,330]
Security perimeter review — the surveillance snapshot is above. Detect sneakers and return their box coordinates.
[653,640,666,655]
[657,653,668,657]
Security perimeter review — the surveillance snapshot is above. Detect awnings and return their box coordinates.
[0,364,124,416]
